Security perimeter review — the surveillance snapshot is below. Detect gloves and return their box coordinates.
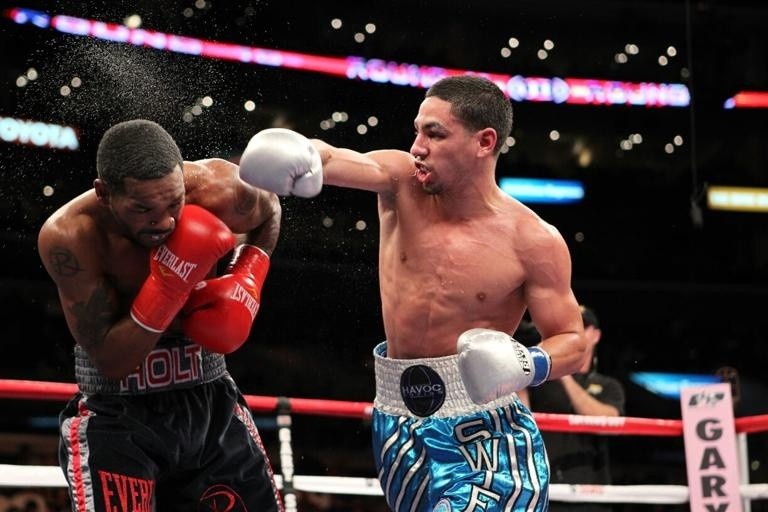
[456,328,553,405]
[238,127,323,198]
[183,242,271,355]
[128,204,238,334]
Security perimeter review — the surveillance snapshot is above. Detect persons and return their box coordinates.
[238,73,589,511]
[38,116,282,509]
[514,305,624,511]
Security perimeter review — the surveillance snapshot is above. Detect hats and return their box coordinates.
[579,305,600,328]
